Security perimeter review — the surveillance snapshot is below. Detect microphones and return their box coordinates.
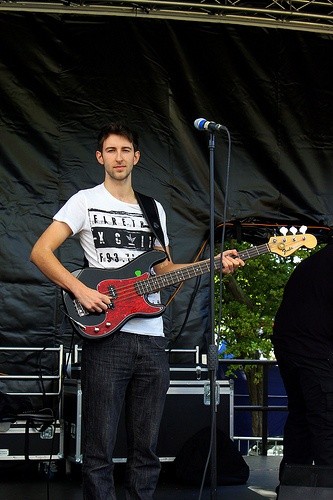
[58,304,86,329]
[193,118,228,134]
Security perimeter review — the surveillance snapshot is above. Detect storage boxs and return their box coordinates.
[72,377,236,470]
[0,375,67,463]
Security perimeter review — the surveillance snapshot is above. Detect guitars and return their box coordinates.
[61,225,317,337]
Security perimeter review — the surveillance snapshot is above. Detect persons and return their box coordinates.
[28,125,245,500]
